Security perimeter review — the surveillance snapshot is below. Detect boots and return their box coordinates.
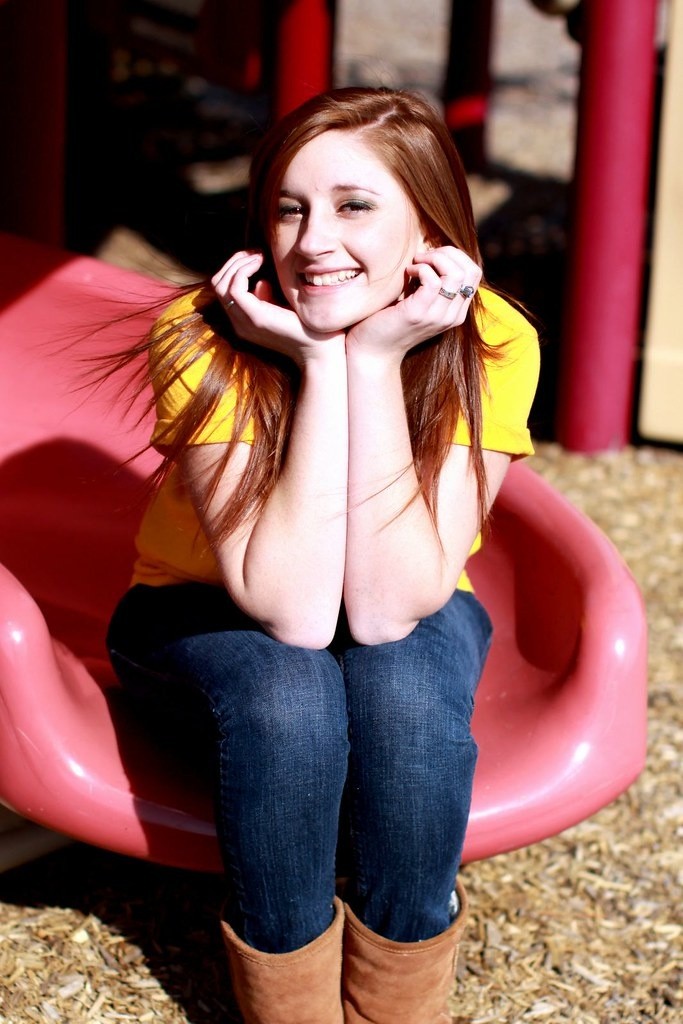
[219,895,343,1024]
[342,875,467,1024]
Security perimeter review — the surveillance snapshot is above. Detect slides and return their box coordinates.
[2,226,655,884]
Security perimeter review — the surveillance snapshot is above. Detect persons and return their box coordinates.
[107,82,539,1024]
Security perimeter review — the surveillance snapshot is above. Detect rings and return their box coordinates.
[439,288,457,299]
[226,301,235,307]
[459,284,474,297]
